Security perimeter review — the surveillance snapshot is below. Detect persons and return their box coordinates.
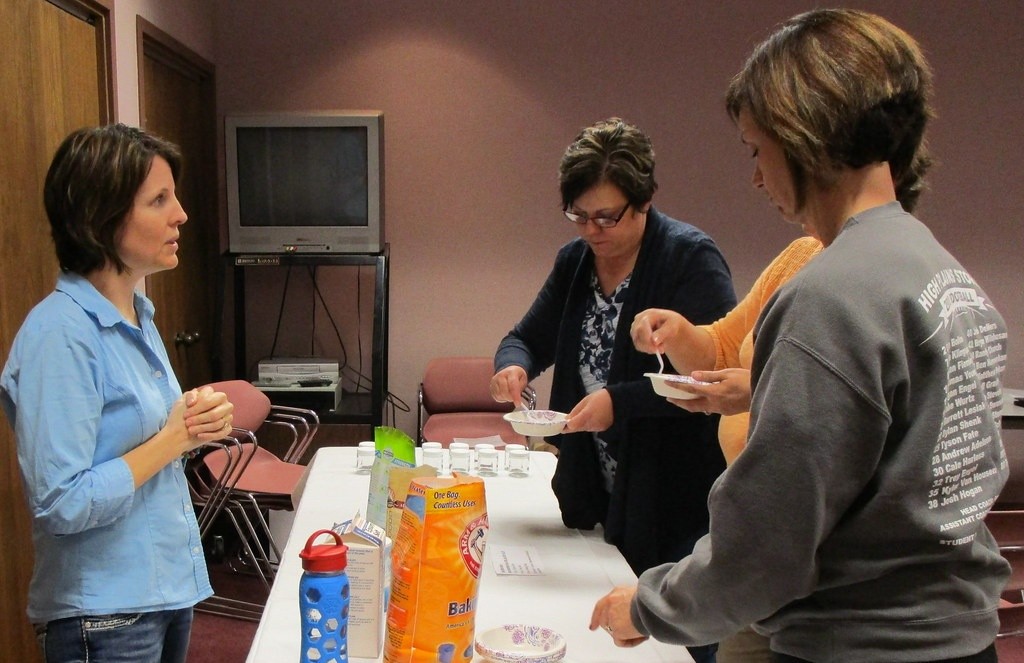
[491,120,737,576]
[1,123,233,663]
[589,11,1011,663]
[630,146,931,663]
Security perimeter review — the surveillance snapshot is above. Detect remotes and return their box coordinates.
[298,379,333,388]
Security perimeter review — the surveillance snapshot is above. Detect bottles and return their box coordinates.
[299,530,350,663]
[356,441,375,471]
[421,442,531,478]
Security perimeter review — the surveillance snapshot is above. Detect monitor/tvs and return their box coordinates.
[225,109,386,254]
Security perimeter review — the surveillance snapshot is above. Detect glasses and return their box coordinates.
[561,198,636,229]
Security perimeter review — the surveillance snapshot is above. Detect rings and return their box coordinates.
[220,417,228,431]
[606,624,612,632]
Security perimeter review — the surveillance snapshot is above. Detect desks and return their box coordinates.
[246,446,695,663]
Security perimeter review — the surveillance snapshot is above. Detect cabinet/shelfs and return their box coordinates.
[231,244,391,495]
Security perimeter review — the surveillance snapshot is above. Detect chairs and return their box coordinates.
[182,380,320,622]
[417,354,536,450]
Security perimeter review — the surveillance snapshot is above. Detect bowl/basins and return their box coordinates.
[503,410,570,436]
[474,624,567,662]
[643,372,713,400]
